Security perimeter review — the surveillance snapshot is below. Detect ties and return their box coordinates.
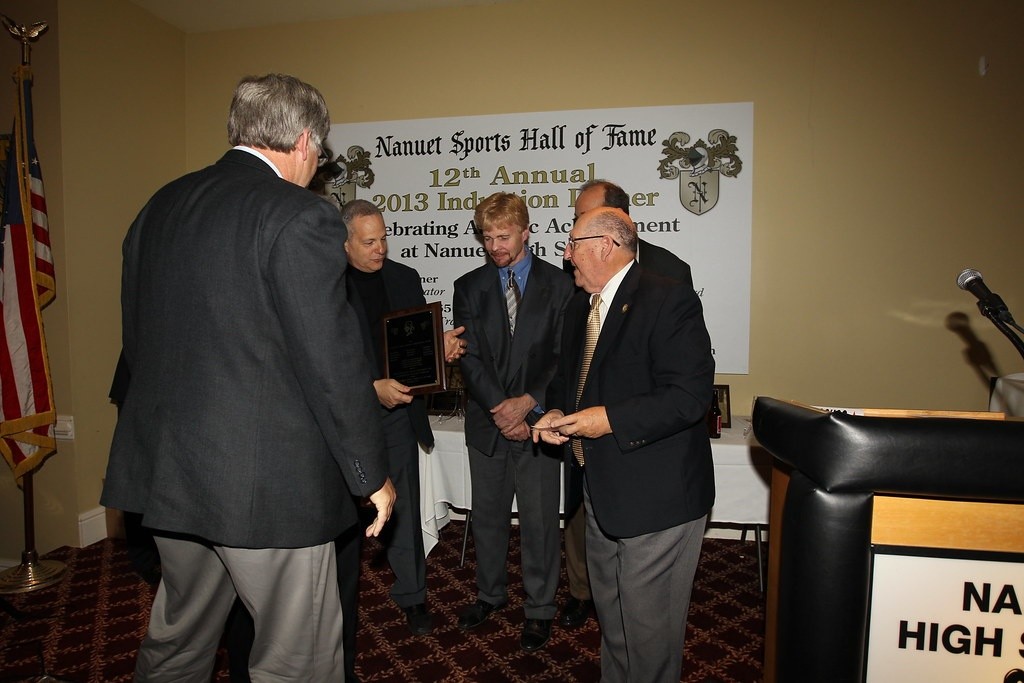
[505,269,521,337]
[573,293,602,467]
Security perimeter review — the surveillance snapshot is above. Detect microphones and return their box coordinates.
[957,268,1015,325]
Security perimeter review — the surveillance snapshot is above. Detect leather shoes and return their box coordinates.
[457,596,505,629]
[561,594,587,628]
[406,603,434,635]
[521,616,551,651]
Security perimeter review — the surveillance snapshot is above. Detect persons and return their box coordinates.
[532,208,716,683]
[451,192,575,651]
[335,199,467,634]
[560,179,695,630]
[100,72,397,683]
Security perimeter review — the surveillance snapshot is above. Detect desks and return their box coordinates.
[419,415,773,593]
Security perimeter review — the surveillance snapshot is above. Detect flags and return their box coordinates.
[0,65,56,489]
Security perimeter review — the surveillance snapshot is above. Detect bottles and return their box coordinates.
[708,389,721,439]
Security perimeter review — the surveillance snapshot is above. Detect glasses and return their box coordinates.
[311,132,330,166]
[569,235,622,250]
